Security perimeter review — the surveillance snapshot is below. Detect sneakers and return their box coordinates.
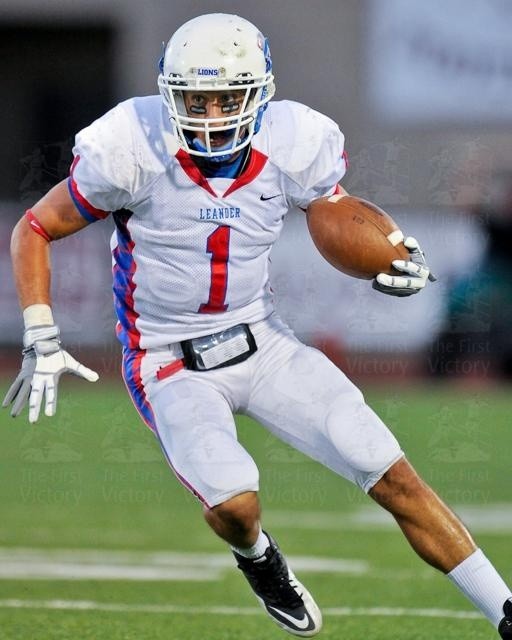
[232,531,322,638]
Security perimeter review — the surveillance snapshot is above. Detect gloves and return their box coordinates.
[372,237,430,296]
[2,303,100,424]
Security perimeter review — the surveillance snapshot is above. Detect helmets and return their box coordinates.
[157,10,277,159]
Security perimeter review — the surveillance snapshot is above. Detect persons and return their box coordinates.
[2,11,512,640]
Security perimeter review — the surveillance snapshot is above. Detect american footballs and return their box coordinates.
[306,195,411,281]
